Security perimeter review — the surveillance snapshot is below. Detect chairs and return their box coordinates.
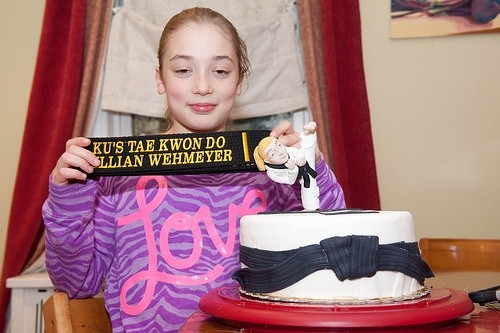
[419,238,500,274]
[41,292,112,333]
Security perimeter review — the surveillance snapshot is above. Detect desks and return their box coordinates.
[5,273,55,333]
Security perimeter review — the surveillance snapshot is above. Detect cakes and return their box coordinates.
[232,208,435,301]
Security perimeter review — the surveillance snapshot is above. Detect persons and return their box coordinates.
[254,120,320,212]
[42,8,346,332]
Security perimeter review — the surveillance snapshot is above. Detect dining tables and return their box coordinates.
[180,309,500,333]
